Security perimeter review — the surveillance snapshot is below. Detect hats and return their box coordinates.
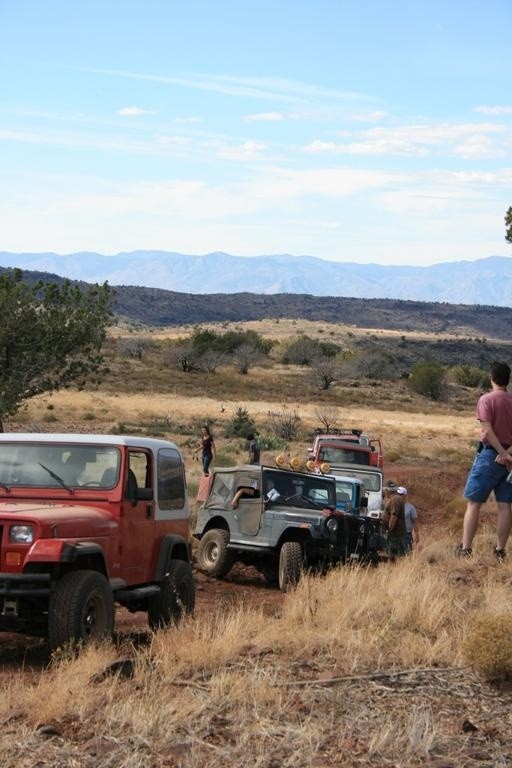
[382,479,396,487]
[397,487,408,494]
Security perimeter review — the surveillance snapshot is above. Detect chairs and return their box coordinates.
[362,478,373,490]
[334,492,351,502]
[27,462,78,487]
[99,468,140,497]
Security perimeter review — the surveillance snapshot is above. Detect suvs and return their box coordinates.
[0,431,200,663]
[308,424,385,555]
[193,453,385,596]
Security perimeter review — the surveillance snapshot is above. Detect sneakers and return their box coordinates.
[493,545,506,559]
[453,543,472,559]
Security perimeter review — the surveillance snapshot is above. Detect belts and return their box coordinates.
[482,443,509,451]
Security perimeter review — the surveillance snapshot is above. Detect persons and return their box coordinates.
[245,433,261,466]
[191,425,218,477]
[383,486,408,563]
[228,477,277,510]
[389,493,419,552]
[451,361,511,564]
